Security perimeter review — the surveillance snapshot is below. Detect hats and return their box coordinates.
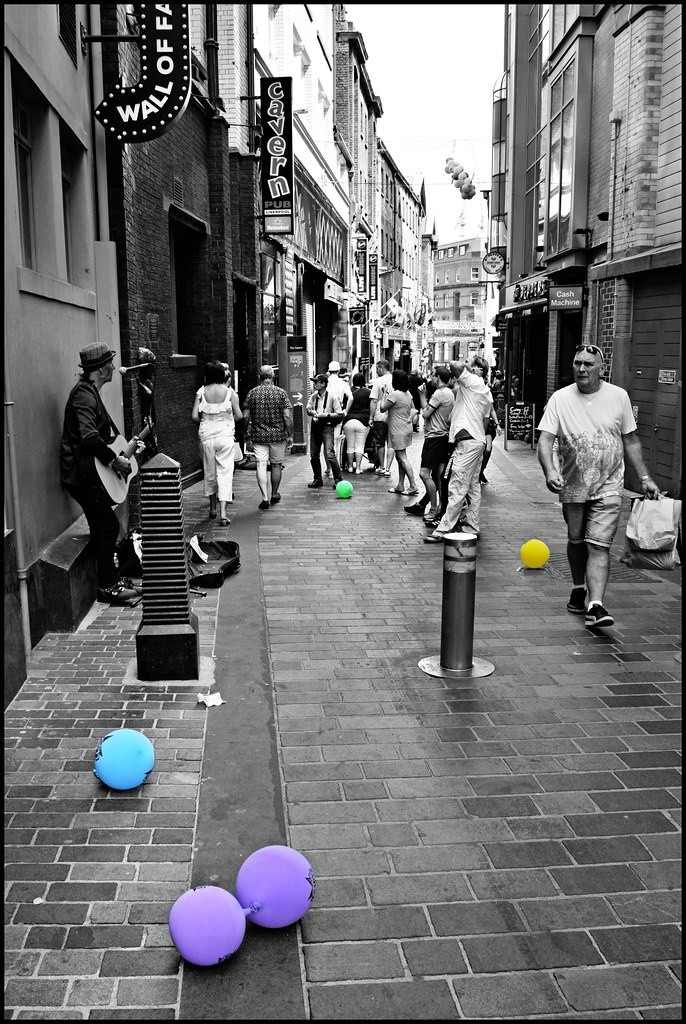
[261,365,274,375]
[310,374,328,382]
[328,361,340,371]
[77,342,116,368]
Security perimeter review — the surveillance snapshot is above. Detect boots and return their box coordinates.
[479,447,491,483]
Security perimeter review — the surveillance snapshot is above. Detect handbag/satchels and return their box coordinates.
[619,494,681,570]
[234,441,243,462]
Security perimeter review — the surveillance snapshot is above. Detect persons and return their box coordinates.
[305,373,345,488]
[192,360,243,526]
[325,356,506,542]
[242,365,293,509]
[537,344,660,626]
[59,342,145,601]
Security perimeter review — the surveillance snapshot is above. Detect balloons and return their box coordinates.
[520,538,550,569]
[445,158,475,200]
[92,729,155,790]
[168,886,254,967]
[336,480,353,498]
[235,845,315,929]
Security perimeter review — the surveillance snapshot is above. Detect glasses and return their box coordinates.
[576,345,603,363]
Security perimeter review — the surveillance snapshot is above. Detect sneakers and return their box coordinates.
[567,587,587,612]
[584,604,614,626]
[97,584,137,603]
[118,581,142,594]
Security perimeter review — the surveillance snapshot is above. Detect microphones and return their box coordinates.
[119,363,150,375]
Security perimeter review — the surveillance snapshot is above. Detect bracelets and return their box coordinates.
[639,475,650,483]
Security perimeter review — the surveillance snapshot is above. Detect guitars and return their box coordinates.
[92,415,157,506]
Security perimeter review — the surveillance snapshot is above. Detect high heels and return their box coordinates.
[210,511,217,518]
[222,518,230,525]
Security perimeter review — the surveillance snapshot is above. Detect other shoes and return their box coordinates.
[345,466,480,542]
[270,493,281,502]
[308,479,323,488]
[259,501,269,509]
[333,479,340,488]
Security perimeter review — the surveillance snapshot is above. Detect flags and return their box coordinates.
[388,298,435,333]
